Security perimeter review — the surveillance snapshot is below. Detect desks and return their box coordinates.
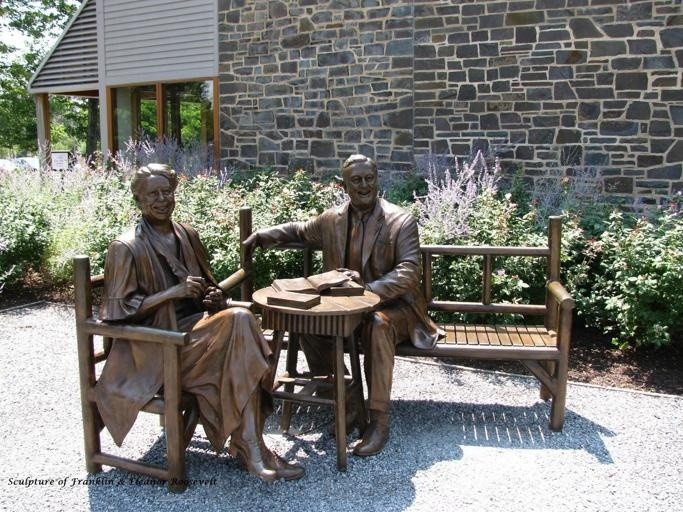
[248,279,382,474]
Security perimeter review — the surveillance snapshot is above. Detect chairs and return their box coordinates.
[69,247,255,494]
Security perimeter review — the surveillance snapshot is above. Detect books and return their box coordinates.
[267,269,351,296]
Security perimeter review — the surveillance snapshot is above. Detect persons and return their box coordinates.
[94,162,304,483]
[243,153,447,456]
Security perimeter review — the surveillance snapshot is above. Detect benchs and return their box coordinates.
[234,201,579,436]
